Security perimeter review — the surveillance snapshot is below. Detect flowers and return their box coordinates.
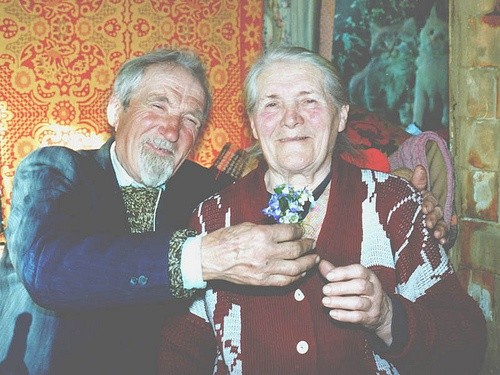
[261,182,314,225]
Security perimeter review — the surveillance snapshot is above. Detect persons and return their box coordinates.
[0,49,449,375]
[155,47,488,375]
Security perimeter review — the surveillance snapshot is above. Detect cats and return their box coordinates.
[348,4,449,128]
